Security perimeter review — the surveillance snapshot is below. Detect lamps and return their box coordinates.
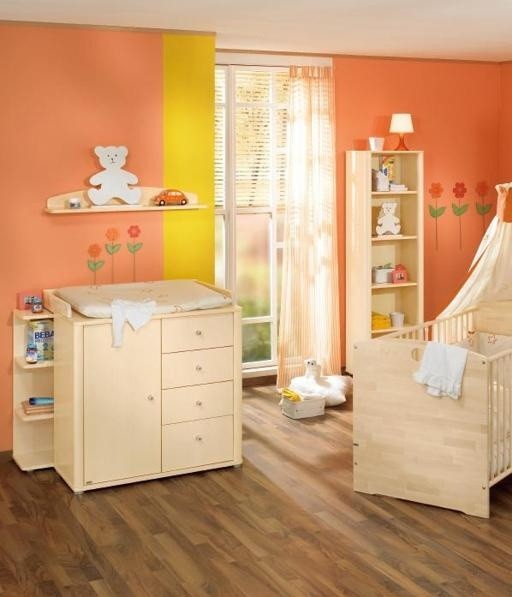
[388,112,416,151]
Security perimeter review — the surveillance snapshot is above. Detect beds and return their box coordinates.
[344,295,512,518]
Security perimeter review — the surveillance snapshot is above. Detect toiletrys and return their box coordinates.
[30,397,54,406]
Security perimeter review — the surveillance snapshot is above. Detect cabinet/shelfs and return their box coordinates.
[8,278,249,496]
[342,148,429,375]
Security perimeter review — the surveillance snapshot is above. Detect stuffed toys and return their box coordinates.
[305,358,323,381]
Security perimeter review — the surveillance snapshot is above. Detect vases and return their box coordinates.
[370,136,385,150]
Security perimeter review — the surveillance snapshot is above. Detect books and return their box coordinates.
[390,188,408,191]
[22,399,55,409]
[21,406,54,414]
[389,184,406,188]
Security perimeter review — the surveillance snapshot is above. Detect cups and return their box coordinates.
[369,138,384,151]
[390,311,405,329]
